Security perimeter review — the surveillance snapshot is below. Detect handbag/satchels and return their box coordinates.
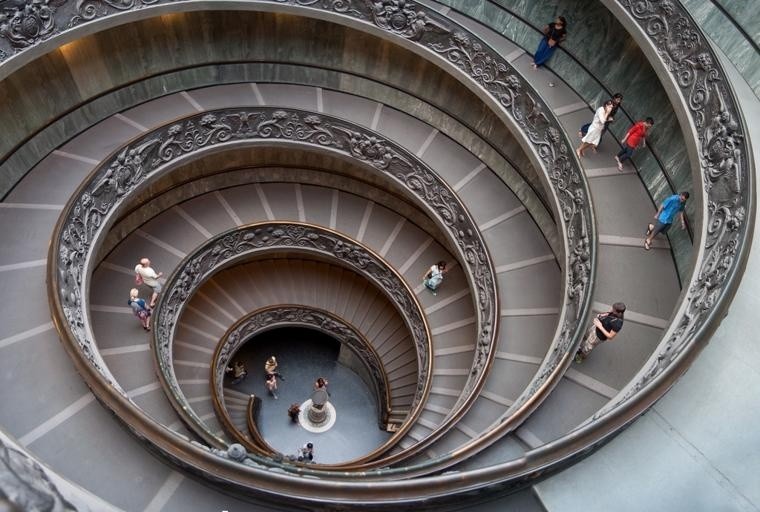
[135,274,143,284]
[428,273,432,279]
[581,123,592,137]
[138,310,148,320]
[548,39,556,48]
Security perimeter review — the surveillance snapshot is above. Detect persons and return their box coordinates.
[531,16,567,70]
[225,355,286,399]
[575,302,626,363]
[313,375,331,396]
[298,441,314,461]
[288,402,300,423]
[127,258,164,330]
[645,192,689,250]
[422,260,448,296]
[577,93,654,172]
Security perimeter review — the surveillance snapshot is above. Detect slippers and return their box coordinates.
[646,223,652,235]
[644,238,652,249]
